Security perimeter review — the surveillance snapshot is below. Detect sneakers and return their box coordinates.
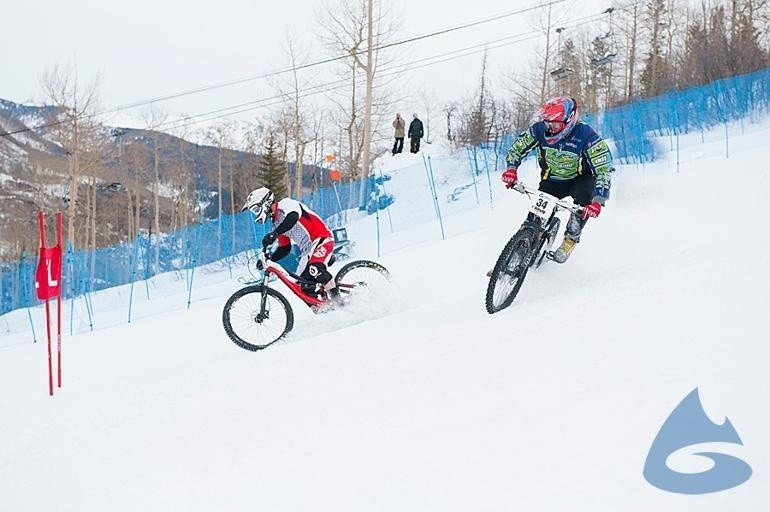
[553,236,577,264]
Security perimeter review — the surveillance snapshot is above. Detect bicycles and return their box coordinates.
[482,177,576,315]
[219,244,396,349]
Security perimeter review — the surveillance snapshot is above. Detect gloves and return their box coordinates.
[501,170,518,189]
[261,232,277,248]
[580,201,600,221]
[255,257,264,271]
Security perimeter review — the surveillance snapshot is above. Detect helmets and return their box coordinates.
[540,96,579,146]
[238,185,275,226]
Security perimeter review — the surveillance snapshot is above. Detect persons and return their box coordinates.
[392,112,406,154]
[502,96,615,264]
[408,113,423,153]
[241,186,343,315]
